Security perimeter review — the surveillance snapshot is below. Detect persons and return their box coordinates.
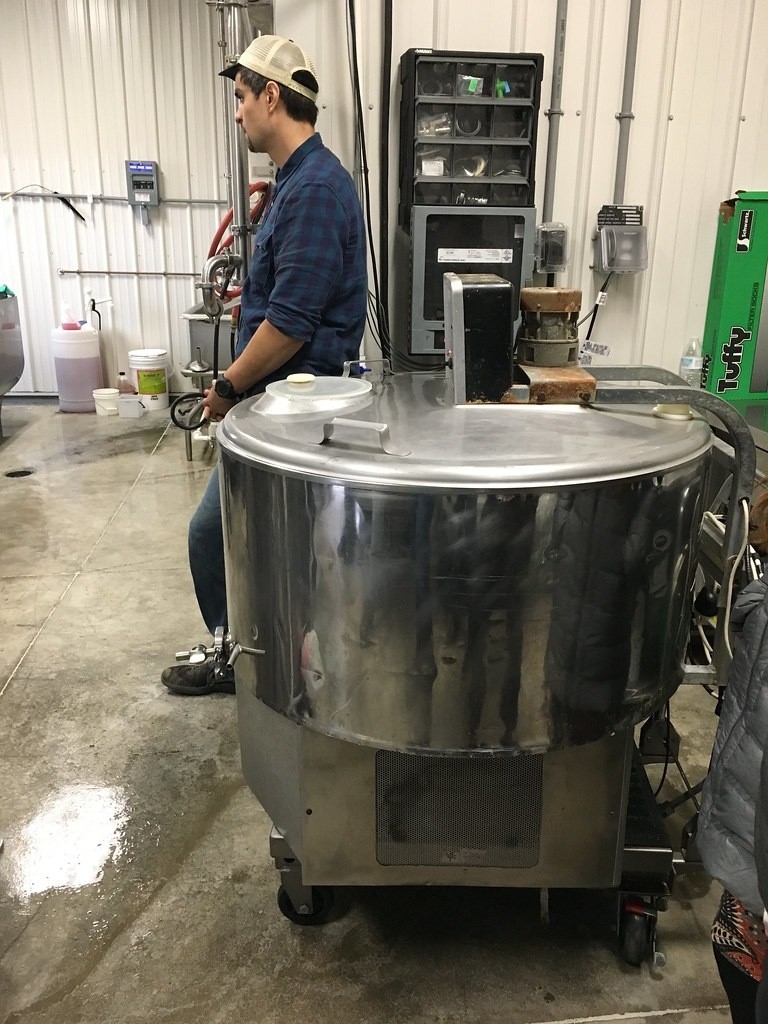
[157,33,371,699]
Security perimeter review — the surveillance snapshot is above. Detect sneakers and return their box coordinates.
[162,656,235,695]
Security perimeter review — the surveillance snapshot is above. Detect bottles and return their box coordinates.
[116,371,129,393]
[679,336,702,389]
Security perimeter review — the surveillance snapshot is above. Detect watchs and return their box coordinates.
[215,374,241,400]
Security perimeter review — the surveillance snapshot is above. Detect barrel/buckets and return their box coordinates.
[50,320,104,412]
[117,395,145,418]
[92,388,120,416]
[128,348,169,411]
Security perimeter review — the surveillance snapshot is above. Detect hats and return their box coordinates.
[218,35,319,103]
